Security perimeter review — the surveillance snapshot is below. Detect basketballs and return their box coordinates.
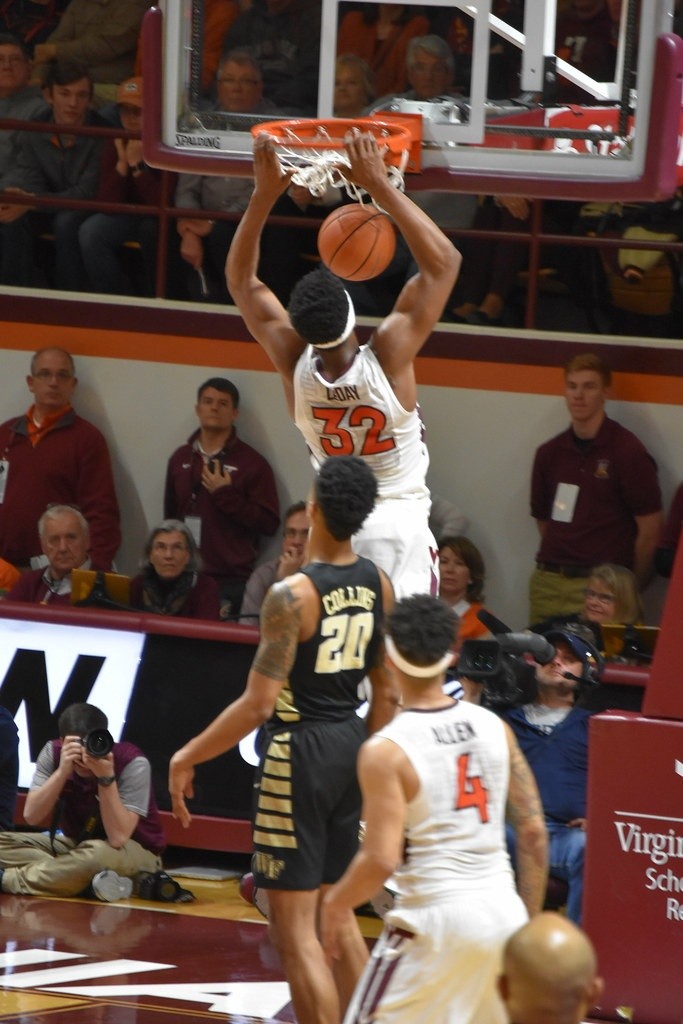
[317,203,395,282]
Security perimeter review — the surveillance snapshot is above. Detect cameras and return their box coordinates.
[76,729,114,756]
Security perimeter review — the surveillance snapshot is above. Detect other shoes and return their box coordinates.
[92,869,134,902]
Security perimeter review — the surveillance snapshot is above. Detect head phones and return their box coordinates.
[566,632,603,695]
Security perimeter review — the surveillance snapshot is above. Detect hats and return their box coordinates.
[544,623,596,663]
[117,76,144,108]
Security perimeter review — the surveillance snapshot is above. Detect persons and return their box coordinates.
[432,536,503,642]
[562,564,651,712]
[169,454,403,1023]
[0,703,165,904]
[226,130,465,609]
[162,378,282,617]
[8,503,91,606]
[130,520,221,624]
[237,501,311,625]
[528,354,664,624]
[493,915,605,1024]
[457,620,610,925]
[0,346,122,571]
[318,596,550,1024]
[0,0,682,339]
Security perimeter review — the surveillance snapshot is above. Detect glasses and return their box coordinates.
[585,589,615,605]
[34,371,71,380]
[118,105,142,116]
[284,527,309,539]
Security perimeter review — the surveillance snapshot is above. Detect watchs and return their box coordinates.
[96,775,116,788]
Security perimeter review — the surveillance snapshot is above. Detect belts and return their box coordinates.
[536,561,608,577]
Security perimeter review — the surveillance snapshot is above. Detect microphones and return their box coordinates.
[564,671,601,688]
[477,609,513,636]
